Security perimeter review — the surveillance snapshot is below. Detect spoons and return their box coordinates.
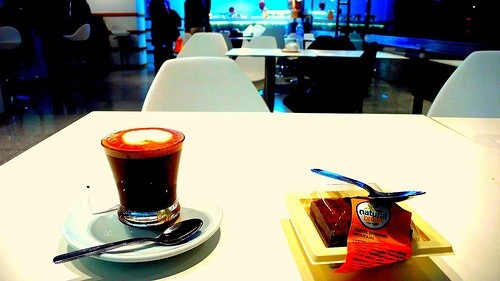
[312,168,426,201]
[53,218,204,264]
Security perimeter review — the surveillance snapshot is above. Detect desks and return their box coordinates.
[0,32,500,281]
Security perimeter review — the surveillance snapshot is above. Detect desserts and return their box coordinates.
[309,195,413,248]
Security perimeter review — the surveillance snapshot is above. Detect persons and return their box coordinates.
[259,2,267,16]
[184,0,212,34]
[149,0,182,72]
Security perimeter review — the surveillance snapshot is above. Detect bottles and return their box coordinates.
[296,22,304,50]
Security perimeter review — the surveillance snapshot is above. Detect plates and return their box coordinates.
[282,47,298,53]
[61,194,225,263]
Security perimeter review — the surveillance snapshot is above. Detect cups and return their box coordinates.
[100,127,186,228]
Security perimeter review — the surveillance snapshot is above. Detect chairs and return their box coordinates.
[234,34,278,83]
[428,47,500,117]
[176,33,227,58]
[283,35,368,113]
[139,55,273,113]
